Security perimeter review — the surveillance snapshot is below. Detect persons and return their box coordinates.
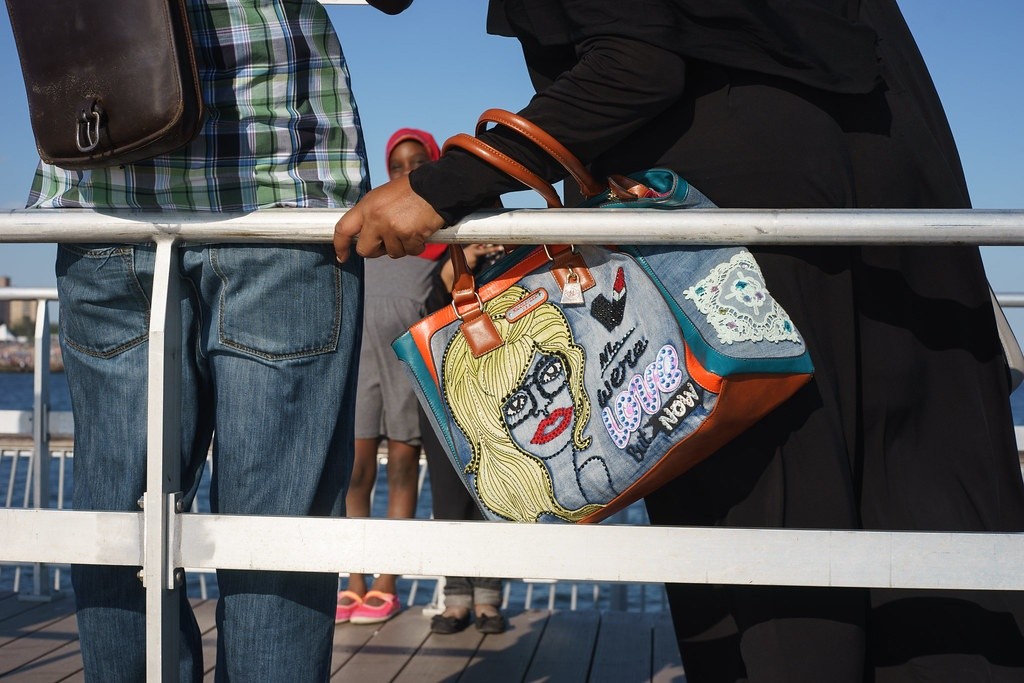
[334,126,523,634]
[334,1,1024,682]
[4,0,416,683]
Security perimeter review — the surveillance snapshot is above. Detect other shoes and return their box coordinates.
[335,591,366,623]
[476,605,506,632]
[349,590,401,623]
[431,608,471,633]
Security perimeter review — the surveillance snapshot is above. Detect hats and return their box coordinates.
[385,128,438,175]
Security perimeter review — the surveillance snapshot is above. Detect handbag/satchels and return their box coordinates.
[5,0,203,171]
[390,109,815,526]
